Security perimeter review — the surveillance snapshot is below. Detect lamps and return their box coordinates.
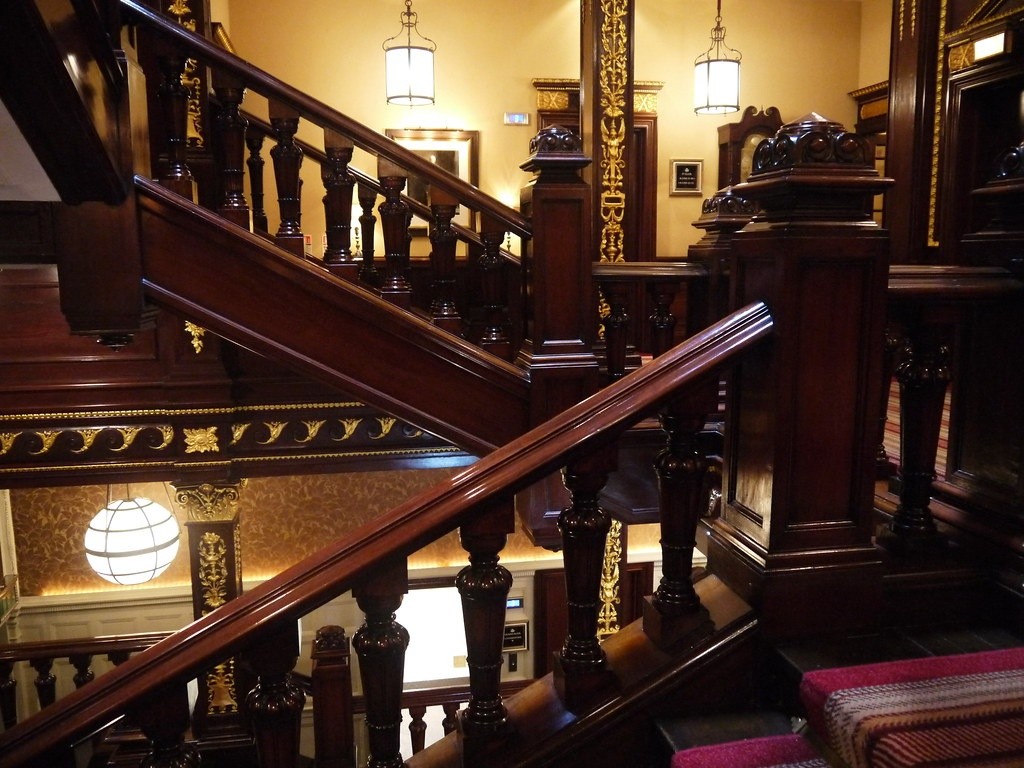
[694,0,742,115]
[382,0,437,107]
[84,483,182,586]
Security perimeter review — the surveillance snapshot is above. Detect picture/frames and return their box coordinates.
[385,129,480,237]
[668,159,705,197]
